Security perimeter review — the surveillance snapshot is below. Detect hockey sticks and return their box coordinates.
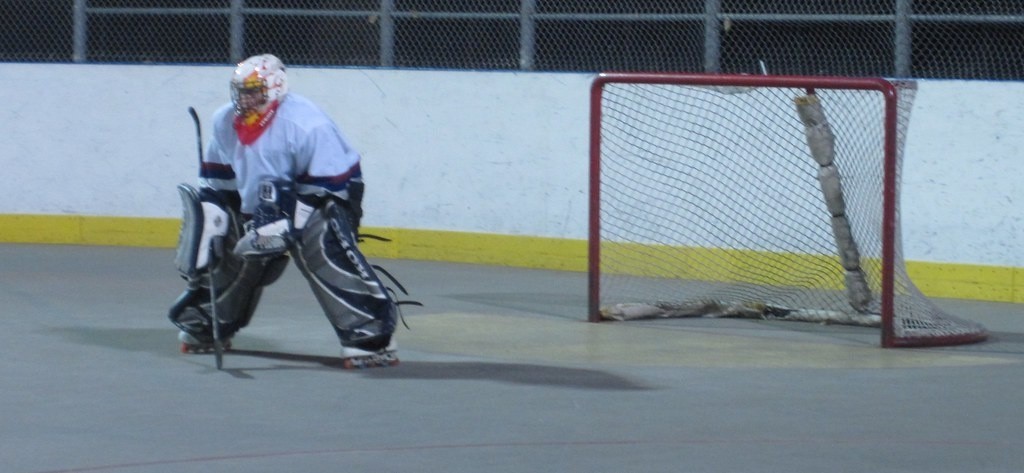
[188,105,225,371]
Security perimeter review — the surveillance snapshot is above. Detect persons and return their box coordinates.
[168,50,404,371]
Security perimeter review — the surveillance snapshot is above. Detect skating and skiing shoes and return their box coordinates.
[343,343,399,371]
[178,332,232,354]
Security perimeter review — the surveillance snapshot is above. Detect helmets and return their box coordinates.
[228,53,289,147]
